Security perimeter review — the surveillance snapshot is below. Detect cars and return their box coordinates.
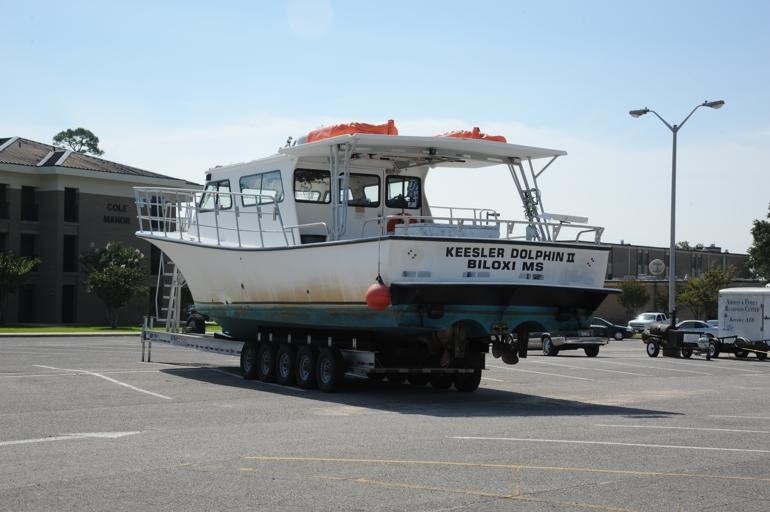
[502,324,611,357]
[674,318,719,338]
[586,316,634,341]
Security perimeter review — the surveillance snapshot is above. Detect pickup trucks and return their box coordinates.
[628,313,679,334]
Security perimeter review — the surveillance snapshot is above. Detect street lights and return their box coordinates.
[629,100,725,325]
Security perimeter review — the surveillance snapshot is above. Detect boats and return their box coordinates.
[132,119,624,366]
